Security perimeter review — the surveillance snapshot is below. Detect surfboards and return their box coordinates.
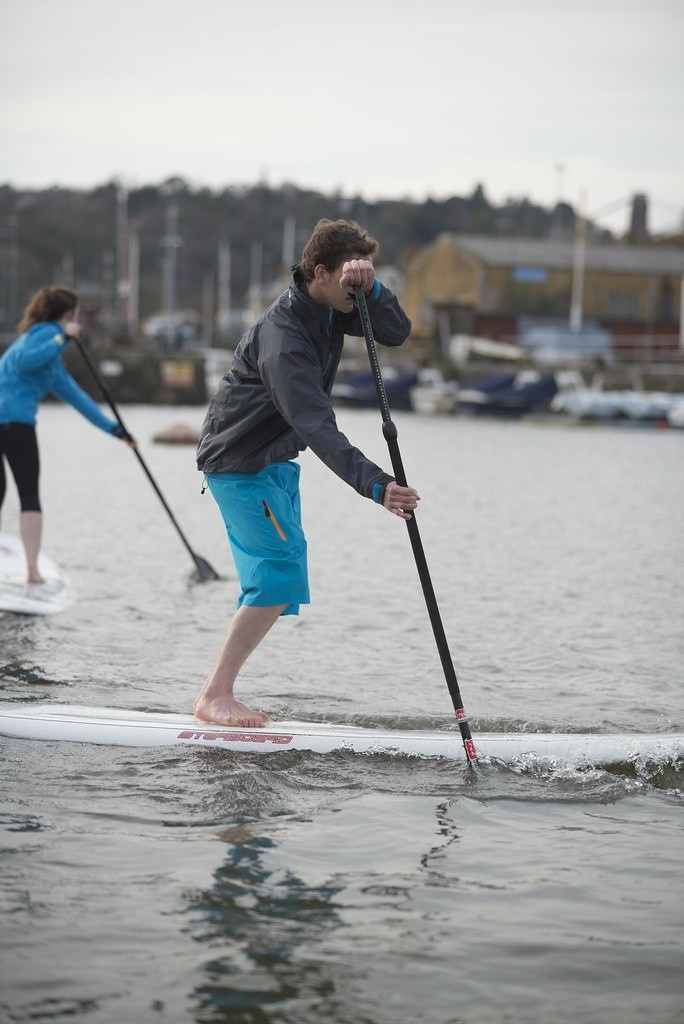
[0,530,73,617]
[1,694,681,767]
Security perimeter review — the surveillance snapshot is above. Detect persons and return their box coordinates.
[0,284,136,586]
[192,218,421,727]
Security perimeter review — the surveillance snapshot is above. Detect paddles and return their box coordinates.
[70,333,217,581]
[350,278,483,764]
